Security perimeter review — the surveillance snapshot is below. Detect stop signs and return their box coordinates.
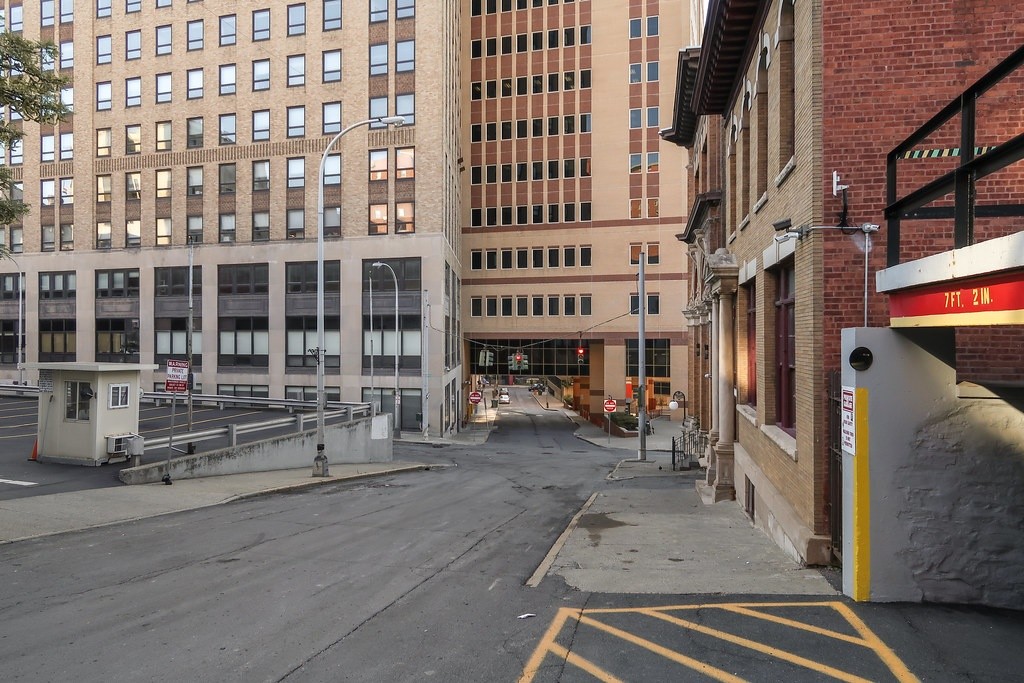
[603,400,616,412]
[469,392,482,404]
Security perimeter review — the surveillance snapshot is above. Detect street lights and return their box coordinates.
[306,117,404,477]
[0,250,23,396]
[372,261,400,438]
[669,391,686,445]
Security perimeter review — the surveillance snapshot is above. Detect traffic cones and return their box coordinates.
[28,440,38,461]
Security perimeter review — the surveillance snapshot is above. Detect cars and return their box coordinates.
[498,388,510,404]
[529,383,542,391]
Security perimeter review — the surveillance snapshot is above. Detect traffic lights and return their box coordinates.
[577,347,585,365]
[486,352,494,366]
[508,356,515,370]
[516,354,523,370]
[523,355,528,369]
